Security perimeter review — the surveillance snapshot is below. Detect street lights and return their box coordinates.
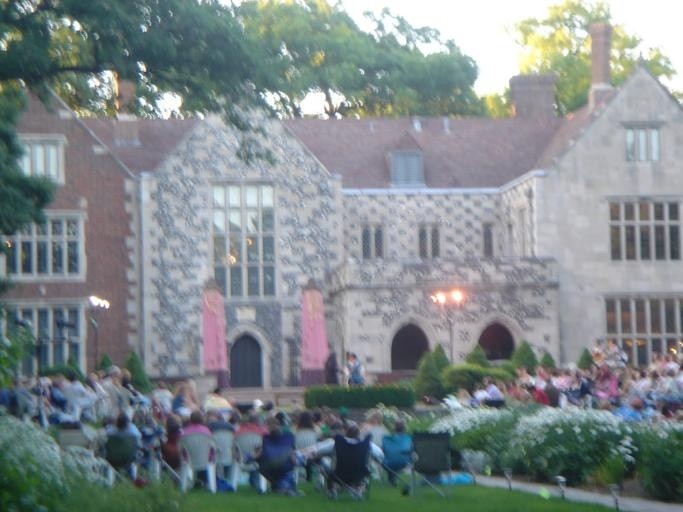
[83,292,113,372]
[431,289,466,367]
[53,318,76,364]
[12,315,33,418]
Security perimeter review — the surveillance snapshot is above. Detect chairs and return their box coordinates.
[98,429,455,504]
[483,398,506,410]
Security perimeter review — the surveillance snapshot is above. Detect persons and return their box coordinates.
[592,339,628,361]
[0,365,231,429]
[325,353,366,386]
[459,353,683,425]
[100,399,414,494]
